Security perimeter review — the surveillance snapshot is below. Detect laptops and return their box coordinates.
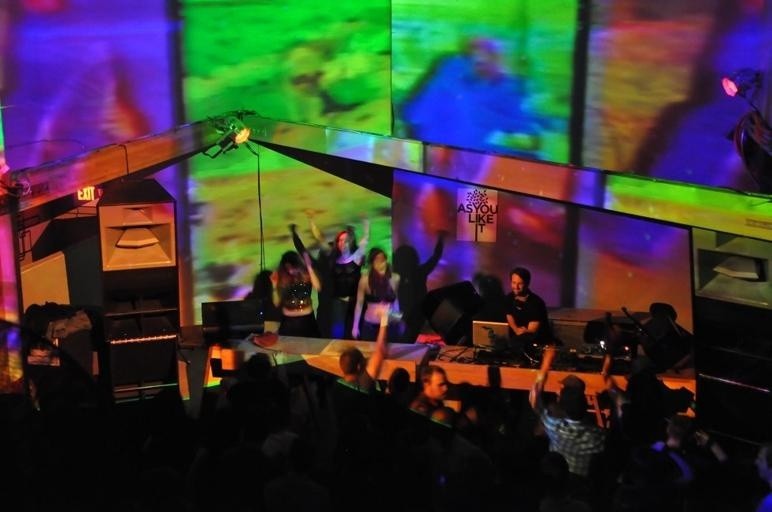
[471,320,510,348]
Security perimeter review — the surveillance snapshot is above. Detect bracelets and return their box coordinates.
[272,287,277,291]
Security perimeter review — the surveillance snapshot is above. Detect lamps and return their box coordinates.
[212,114,250,151]
[720,67,762,115]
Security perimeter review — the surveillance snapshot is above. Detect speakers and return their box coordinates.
[94,176,179,403]
[689,225,772,444]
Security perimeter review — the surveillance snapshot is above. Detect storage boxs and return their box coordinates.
[546,306,654,350]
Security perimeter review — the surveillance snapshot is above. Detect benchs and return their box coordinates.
[201,299,278,345]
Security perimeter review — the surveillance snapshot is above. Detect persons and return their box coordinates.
[304,207,370,337]
[270,251,324,337]
[503,268,552,367]
[137,340,730,510]
[351,248,401,342]
[751,440,772,511]
